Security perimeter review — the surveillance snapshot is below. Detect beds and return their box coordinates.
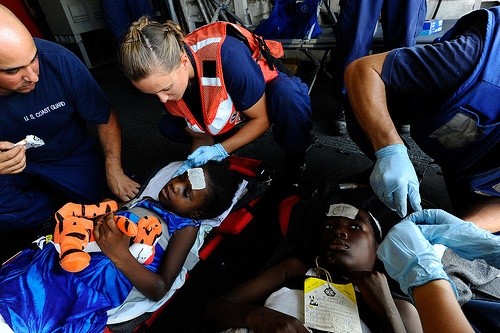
[0,156,275,333]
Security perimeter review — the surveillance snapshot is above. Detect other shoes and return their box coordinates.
[274,147,311,198]
[332,102,354,134]
[396,118,411,134]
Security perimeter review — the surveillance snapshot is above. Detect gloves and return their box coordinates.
[369,144,422,218]
[376,220,459,303]
[400,207,500,269]
[187,143,230,167]
[171,160,193,179]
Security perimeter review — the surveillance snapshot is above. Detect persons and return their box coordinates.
[119,15,314,180]
[0,159,243,333]
[376,208,500,333]
[204,202,423,333]
[0,4,141,250]
[103,0,155,85]
[333,0,426,135]
[342,6,500,219]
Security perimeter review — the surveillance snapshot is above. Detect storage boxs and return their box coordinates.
[419,18,443,36]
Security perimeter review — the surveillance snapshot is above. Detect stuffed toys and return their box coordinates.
[53,199,163,273]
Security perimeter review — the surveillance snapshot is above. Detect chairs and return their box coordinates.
[252,0,459,97]
